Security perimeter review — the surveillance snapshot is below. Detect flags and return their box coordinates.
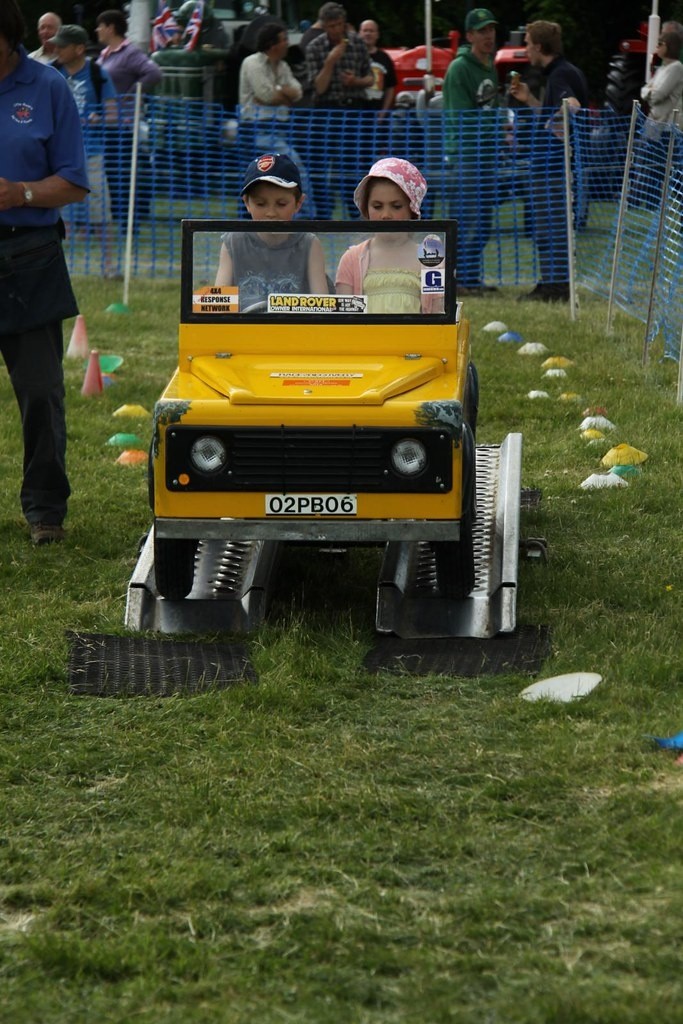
[150,0,204,51]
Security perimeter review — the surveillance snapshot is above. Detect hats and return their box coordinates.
[239,152,303,198]
[466,8,497,32]
[47,23,88,48]
[352,157,429,220]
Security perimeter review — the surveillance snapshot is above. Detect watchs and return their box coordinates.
[22,181,33,208]
[274,83,281,93]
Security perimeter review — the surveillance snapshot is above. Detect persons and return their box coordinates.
[45,24,124,281]
[26,12,62,66]
[442,10,500,295]
[1,0,92,545]
[210,153,333,315]
[238,3,398,219]
[94,10,163,237]
[333,157,446,319]
[511,21,586,301]
[641,30,683,211]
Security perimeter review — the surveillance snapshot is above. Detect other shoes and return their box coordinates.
[454,277,497,294]
[29,518,65,547]
[516,280,570,302]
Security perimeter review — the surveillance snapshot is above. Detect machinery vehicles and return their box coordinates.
[377,31,590,238]
[602,22,667,134]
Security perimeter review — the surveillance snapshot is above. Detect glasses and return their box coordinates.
[658,42,668,47]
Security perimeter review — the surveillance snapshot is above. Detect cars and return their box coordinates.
[148,219,476,599]
[151,0,310,169]
